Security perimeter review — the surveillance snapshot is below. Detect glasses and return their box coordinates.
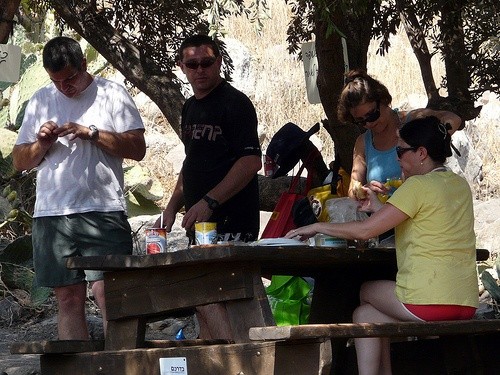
[352,101,380,127]
[396,146,414,159]
[181,58,216,69]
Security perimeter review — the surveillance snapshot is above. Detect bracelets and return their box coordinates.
[89,125,98,138]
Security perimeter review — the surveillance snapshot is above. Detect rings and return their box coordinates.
[196,221,198,222]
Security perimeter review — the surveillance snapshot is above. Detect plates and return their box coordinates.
[257,238,307,245]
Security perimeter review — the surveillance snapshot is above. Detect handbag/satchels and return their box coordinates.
[260,162,313,239]
[306,152,351,222]
[264,275,312,325]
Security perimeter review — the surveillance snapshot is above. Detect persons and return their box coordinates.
[284,116,480,375]
[13,36,146,340]
[337,68,461,203]
[154,34,263,339]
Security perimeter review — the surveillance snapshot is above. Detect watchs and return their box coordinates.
[203,195,219,210]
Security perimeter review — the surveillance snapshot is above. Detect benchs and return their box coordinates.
[249,319,500,340]
[11,339,229,354]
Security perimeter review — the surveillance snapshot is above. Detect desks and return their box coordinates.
[64,243,490,374]
